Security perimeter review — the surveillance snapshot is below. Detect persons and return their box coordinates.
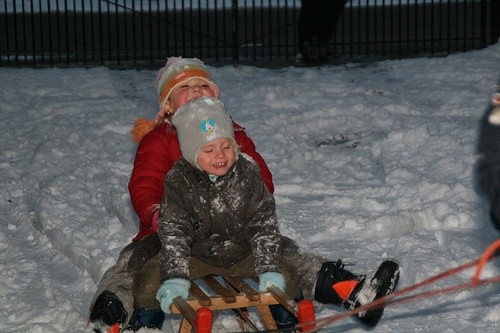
[474,89,500,234]
[125,96,304,333]
[88,56,400,333]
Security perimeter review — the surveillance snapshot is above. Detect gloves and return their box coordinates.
[255,272,287,294]
[156,278,191,314]
[152,210,160,232]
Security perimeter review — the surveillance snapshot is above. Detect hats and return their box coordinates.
[171,95,236,170]
[132,56,218,144]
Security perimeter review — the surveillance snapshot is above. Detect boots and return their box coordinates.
[126,307,166,333]
[312,257,401,327]
[268,290,304,328]
[89,291,128,333]
